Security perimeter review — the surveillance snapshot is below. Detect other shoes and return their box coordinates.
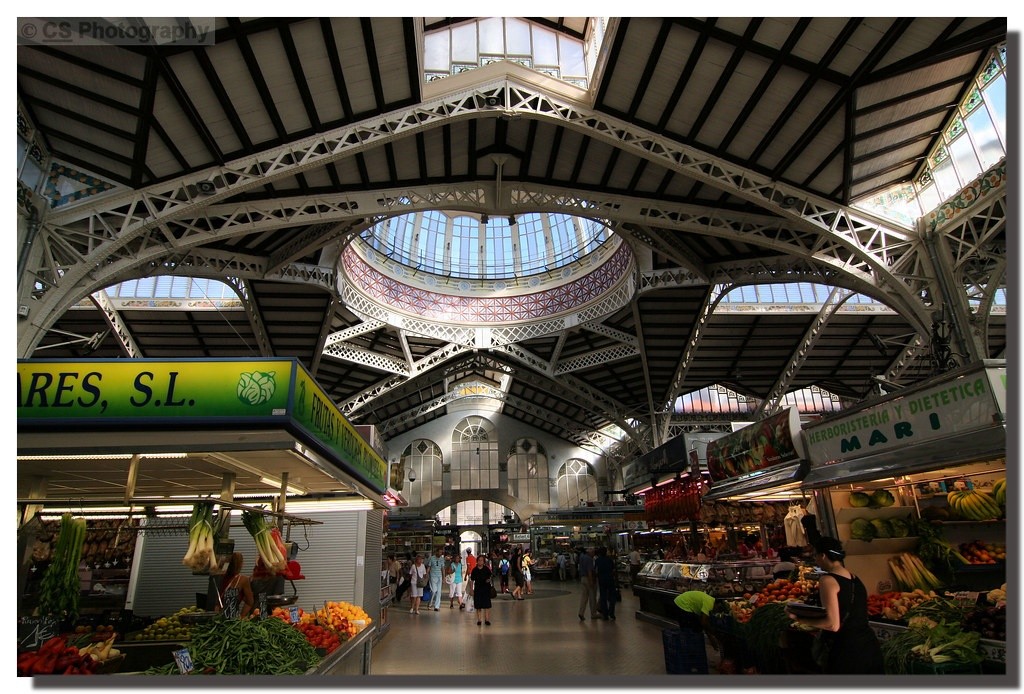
[410,609,413,613]
[518,598,524,600]
[415,611,420,614]
[579,615,585,620]
[460,604,465,609]
[485,621,490,625]
[451,605,453,607]
[477,622,481,625]
[434,609,440,611]
[427,606,431,610]
[591,615,602,619]
[511,592,515,600]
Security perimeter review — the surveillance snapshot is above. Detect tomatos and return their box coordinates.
[293,623,339,656]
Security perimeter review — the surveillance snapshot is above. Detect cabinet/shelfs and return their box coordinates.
[836,506,920,555]
[382,530,434,569]
[934,490,1007,525]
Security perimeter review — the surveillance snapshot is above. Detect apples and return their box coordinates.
[306,615,360,638]
[249,607,303,624]
[137,606,208,639]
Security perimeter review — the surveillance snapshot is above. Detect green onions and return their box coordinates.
[241,504,285,569]
[182,492,221,573]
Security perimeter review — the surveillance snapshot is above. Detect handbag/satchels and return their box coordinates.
[557,564,560,569]
[489,579,497,598]
[390,576,396,583]
[811,632,834,666]
[417,577,427,587]
[445,565,456,584]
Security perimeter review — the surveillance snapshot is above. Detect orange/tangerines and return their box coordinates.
[303,600,372,625]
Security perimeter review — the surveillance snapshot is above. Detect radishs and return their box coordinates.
[78,634,121,662]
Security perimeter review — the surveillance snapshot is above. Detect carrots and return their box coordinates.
[17,637,91,674]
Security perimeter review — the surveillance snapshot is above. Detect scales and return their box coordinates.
[953,589,989,608]
[266,594,298,616]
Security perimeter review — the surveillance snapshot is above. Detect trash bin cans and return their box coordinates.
[101,608,133,642]
[661,627,710,675]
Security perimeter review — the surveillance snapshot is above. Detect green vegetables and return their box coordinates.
[39,513,86,626]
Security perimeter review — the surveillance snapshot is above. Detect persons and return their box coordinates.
[578,547,602,620]
[550,552,567,582]
[625,547,641,584]
[469,555,498,626]
[387,553,412,602]
[783,504,821,547]
[448,555,465,610]
[426,549,446,611]
[499,554,510,594]
[595,547,617,621]
[570,550,601,574]
[213,552,254,620]
[511,547,537,600]
[222,557,230,573]
[464,548,477,599]
[795,535,884,674]
[409,554,427,614]
[651,528,787,562]
[673,591,725,674]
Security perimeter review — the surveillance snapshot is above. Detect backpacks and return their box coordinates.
[502,560,509,575]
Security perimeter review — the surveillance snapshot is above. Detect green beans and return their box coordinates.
[135,616,324,675]
